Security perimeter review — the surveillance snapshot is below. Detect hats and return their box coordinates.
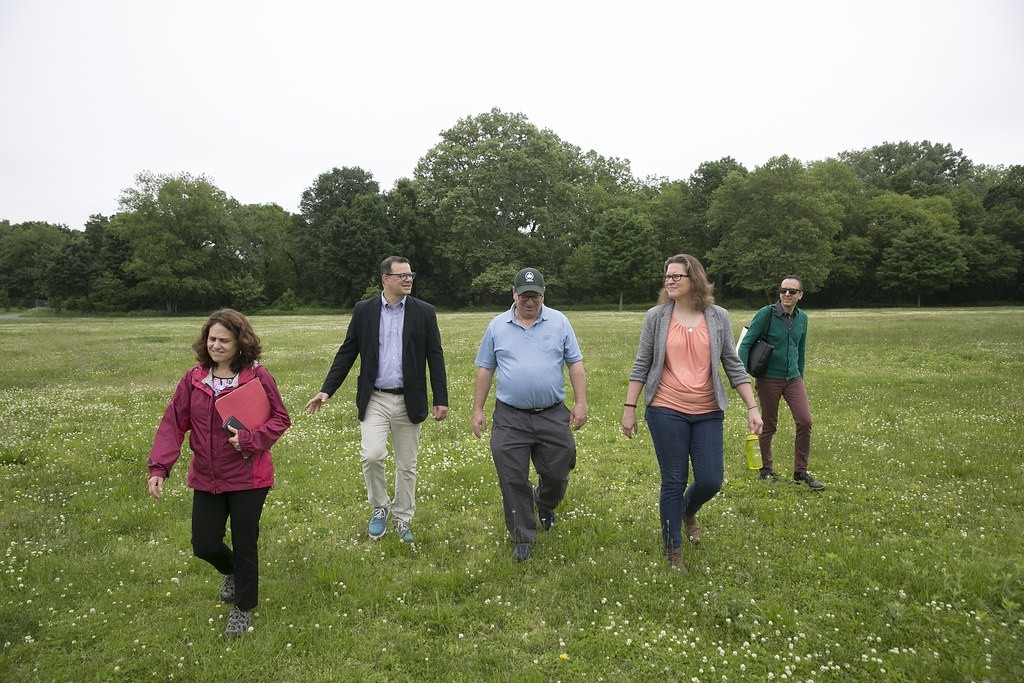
[514,267,545,297]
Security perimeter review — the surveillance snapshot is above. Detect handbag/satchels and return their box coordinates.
[736,306,775,379]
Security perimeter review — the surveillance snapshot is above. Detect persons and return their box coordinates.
[738,276,825,491]
[305,255,449,546]
[145,308,293,635]
[622,254,765,577]
[471,268,588,563]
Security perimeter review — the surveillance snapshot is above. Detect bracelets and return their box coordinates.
[748,405,757,410]
[624,403,637,408]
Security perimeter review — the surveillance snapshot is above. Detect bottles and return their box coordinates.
[746,435,763,470]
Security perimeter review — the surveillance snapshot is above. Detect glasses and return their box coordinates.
[779,287,801,294]
[517,293,543,302]
[380,273,416,281]
[664,273,690,282]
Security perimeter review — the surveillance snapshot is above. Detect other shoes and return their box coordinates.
[666,549,689,576]
[683,515,701,545]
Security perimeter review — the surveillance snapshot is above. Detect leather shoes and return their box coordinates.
[793,471,825,490]
[759,469,782,486]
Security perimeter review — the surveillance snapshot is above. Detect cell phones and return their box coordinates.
[221,415,247,438]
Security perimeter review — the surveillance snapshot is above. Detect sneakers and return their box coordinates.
[534,487,556,530]
[510,541,533,562]
[218,575,238,604]
[394,521,413,542]
[368,503,391,539]
[225,607,253,636]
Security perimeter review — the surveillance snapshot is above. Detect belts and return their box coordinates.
[373,386,404,395]
[497,398,561,414]
